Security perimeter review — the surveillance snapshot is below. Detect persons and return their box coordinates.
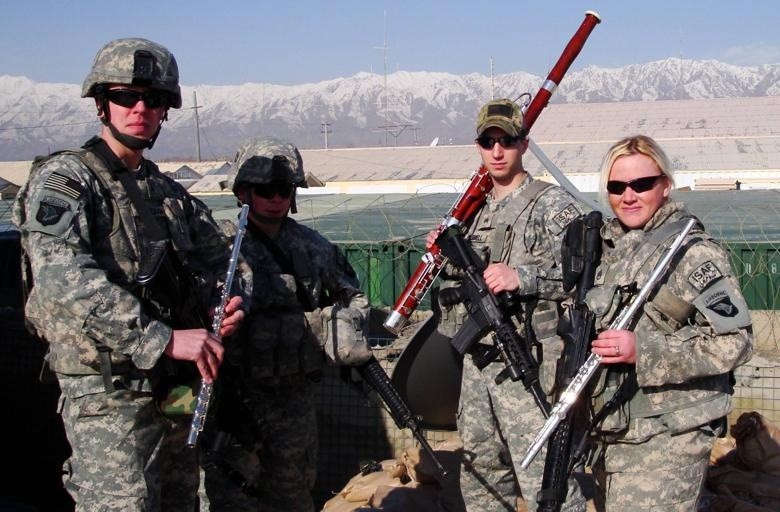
[422,97,604,512]
[197,135,374,511]
[10,36,253,508]
[579,133,755,512]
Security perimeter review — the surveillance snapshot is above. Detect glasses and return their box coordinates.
[606,173,666,196]
[100,87,164,111]
[475,133,522,149]
[251,187,298,200]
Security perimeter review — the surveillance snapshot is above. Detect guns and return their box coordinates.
[536,212,603,510]
[135,240,261,453]
[435,224,555,421]
[290,267,450,481]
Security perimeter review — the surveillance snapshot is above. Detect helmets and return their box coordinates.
[81,35,185,110]
[226,137,304,187]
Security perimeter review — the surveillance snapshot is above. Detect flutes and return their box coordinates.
[186,203,250,446]
[521,218,700,471]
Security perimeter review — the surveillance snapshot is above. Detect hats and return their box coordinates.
[476,97,526,135]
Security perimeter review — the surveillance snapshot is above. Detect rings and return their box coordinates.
[614,346,620,356]
